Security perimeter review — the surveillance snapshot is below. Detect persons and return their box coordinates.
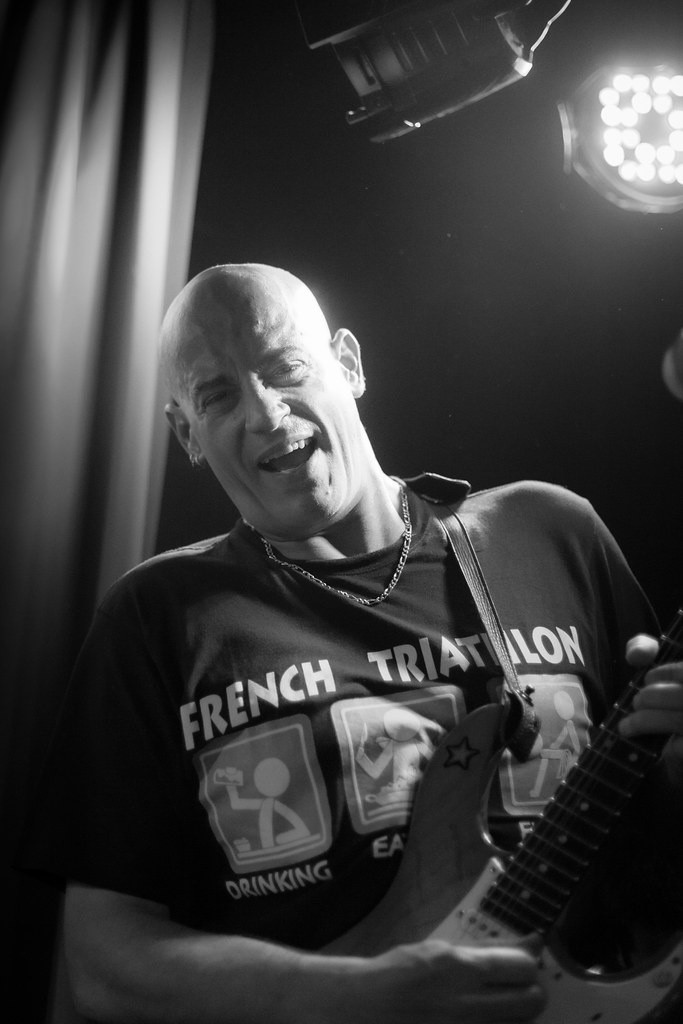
[64,264,683,1024]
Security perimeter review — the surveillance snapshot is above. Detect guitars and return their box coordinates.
[311,606,683,1024]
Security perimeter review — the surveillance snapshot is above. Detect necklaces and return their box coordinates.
[241,475,412,606]
